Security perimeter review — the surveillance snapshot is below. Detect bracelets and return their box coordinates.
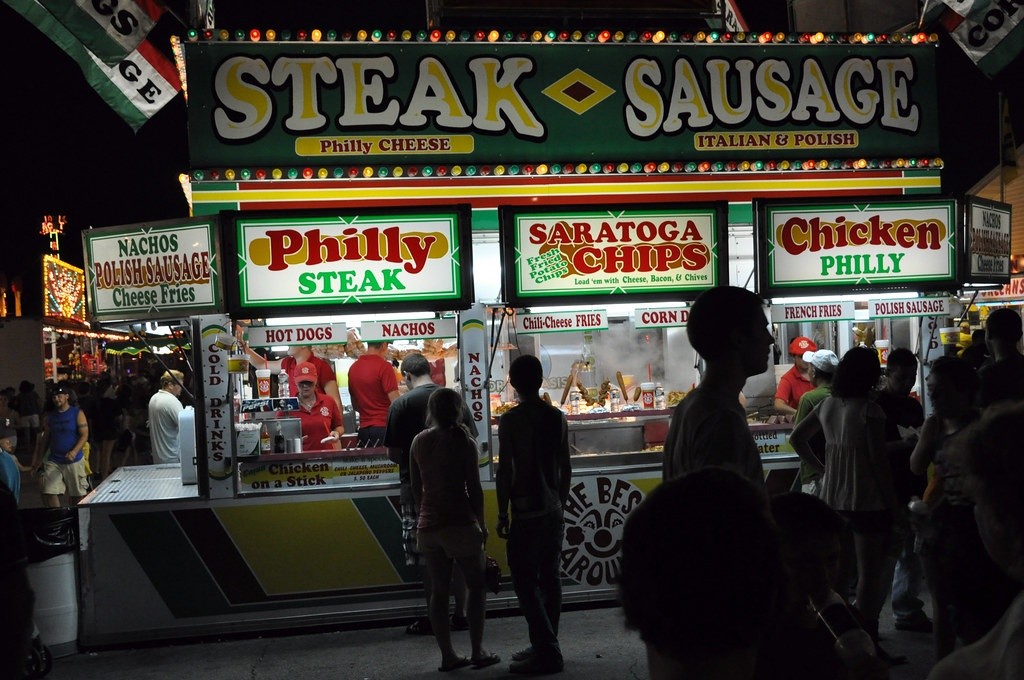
[497,512,509,521]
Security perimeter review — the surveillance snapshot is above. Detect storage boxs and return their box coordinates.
[235,429,261,457]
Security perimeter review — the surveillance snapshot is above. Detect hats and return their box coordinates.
[803,349,838,372]
[295,362,317,384]
[789,337,817,353]
[18,381,34,391]
[1,416,16,438]
[52,388,67,396]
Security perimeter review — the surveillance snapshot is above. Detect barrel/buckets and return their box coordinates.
[227,340,248,374]
[214,320,239,350]
[939,326,960,344]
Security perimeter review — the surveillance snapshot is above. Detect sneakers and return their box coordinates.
[510,645,565,675]
[406,616,469,635]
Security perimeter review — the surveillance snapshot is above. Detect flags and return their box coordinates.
[6,1,183,133]
[929,1,1024,64]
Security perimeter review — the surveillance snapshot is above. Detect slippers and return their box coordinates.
[439,651,499,674]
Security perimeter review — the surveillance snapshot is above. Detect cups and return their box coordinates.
[287,439,301,453]
[641,382,655,410]
[875,340,890,368]
[255,369,271,399]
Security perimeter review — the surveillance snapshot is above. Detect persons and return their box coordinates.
[494,355,573,676]
[346,340,400,449]
[274,365,345,451]
[248,347,280,409]
[406,387,501,670]
[236,334,344,427]
[0,341,194,677]
[619,282,1024,680]
[384,352,479,632]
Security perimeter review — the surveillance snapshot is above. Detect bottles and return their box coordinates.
[276,369,290,398]
[274,421,285,454]
[570,384,581,415]
[261,424,271,455]
[656,383,666,410]
[610,385,620,412]
